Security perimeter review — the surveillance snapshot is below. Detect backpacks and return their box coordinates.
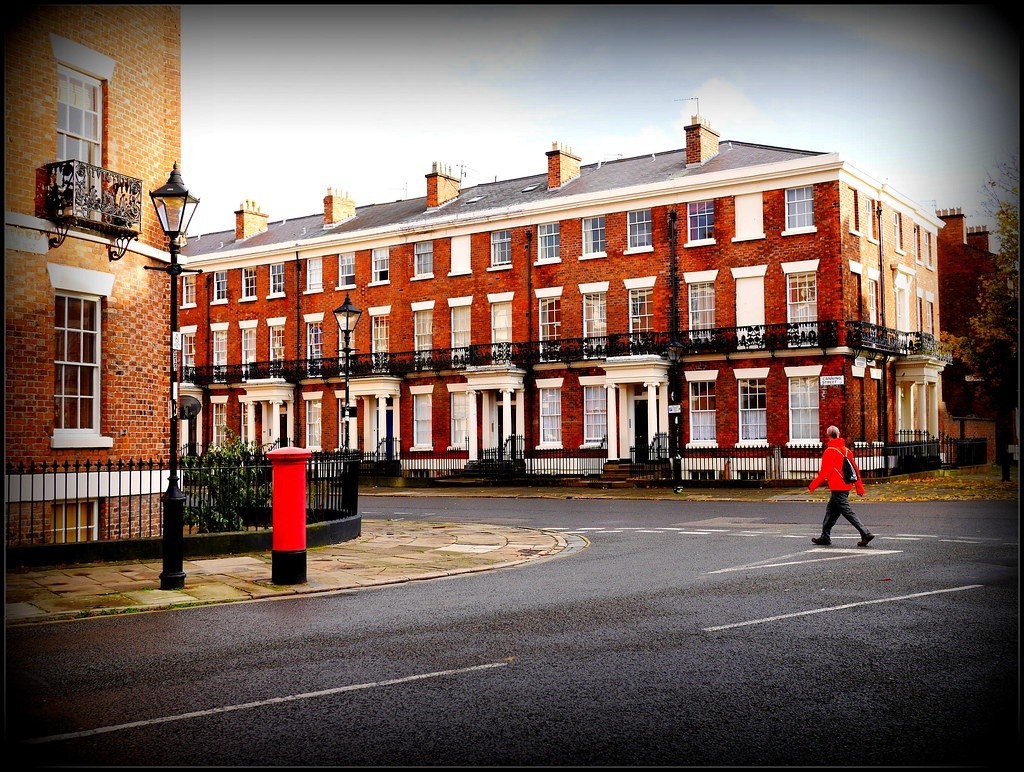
[827,447,858,483]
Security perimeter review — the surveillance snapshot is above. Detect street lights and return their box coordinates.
[332,292,363,454]
[667,342,686,494]
[149,161,202,590]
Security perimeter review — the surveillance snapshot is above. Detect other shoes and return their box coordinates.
[812,534,831,545]
[857,534,874,547]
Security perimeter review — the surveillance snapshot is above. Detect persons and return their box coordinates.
[808,425,874,547]
